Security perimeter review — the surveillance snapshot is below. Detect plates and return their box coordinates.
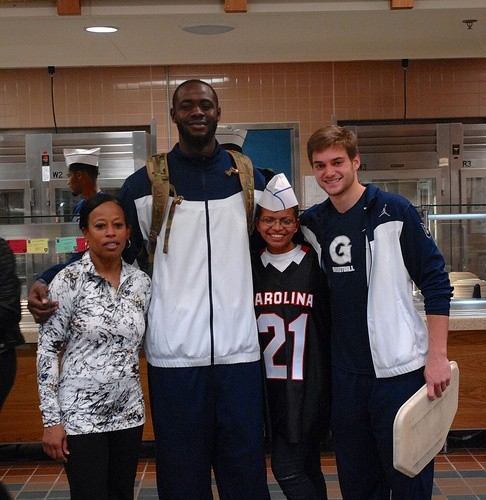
[446,272,486,299]
[19,299,29,312]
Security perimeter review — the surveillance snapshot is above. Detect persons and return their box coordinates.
[0,236,21,500]
[248,173,332,500]
[35,194,152,500]
[215,125,276,184]
[63,148,102,221]
[27,80,273,500]
[298,126,454,500]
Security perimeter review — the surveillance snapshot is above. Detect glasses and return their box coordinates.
[257,217,299,227]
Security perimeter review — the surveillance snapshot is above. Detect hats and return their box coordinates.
[257,172,299,212]
[214,125,247,148]
[63,148,101,167]
